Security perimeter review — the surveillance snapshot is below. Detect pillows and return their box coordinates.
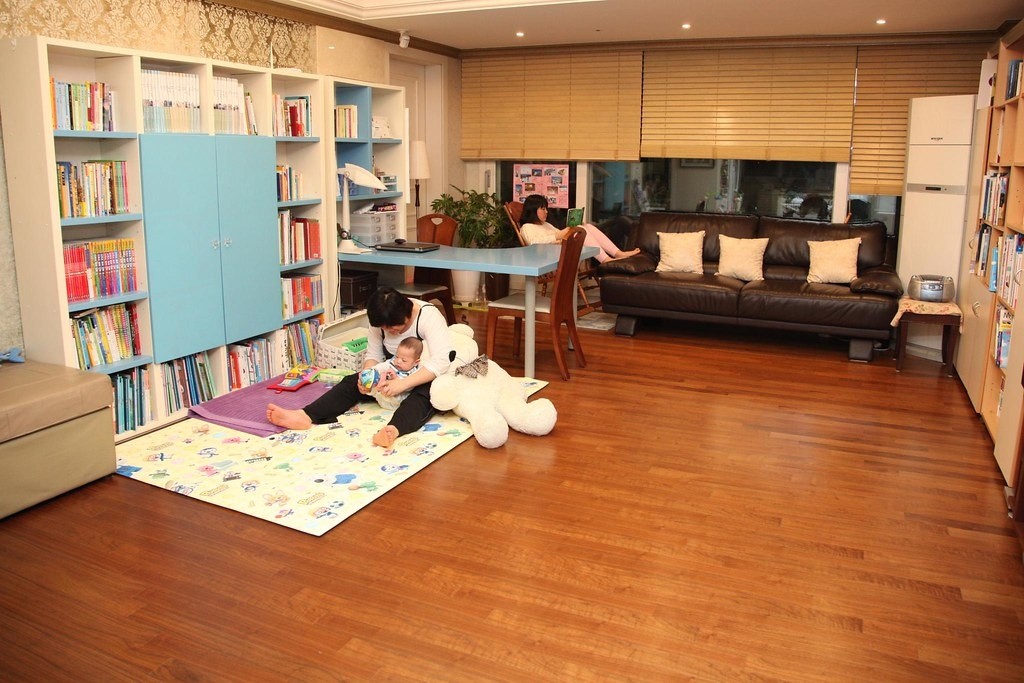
[806,237,861,283]
[655,230,706,275]
[714,234,769,280]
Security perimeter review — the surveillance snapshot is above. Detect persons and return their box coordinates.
[365,337,423,410]
[266,286,451,447]
[520,194,640,264]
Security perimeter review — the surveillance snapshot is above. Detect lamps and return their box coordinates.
[399,29,410,48]
[409,141,431,219]
[337,163,387,254]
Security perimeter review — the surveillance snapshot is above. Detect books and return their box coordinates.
[272,92,312,138]
[276,165,303,202]
[212,76,259,135]
[110,366,154,434]
[50,77,118,132]
[334,104,358,138]
[69,303,142,372]
[283,314,323,369]
[278,208,321,265]
[62,236,138,304]
[266,362,326,392]
[56,159,130,219]
[280,273,322,320]
[141,68,202,133]
[227,337,276,391]
[567,206,585,227]
[162,350,217,417]
[971,59,1023,418]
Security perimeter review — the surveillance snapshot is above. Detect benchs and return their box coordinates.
[0,357,117,519]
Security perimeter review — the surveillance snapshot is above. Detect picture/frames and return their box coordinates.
[680,158,715,169]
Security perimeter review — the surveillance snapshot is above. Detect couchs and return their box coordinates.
[599,213,903,362]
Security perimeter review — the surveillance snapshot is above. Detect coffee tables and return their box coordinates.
[891,295,963,378]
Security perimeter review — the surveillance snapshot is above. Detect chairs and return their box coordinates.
[505,200,601,317]
[486,227,587,380]
[392,214,457,327]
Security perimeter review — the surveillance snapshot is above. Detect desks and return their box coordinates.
[338,241,600,378]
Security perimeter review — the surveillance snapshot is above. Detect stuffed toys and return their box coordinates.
[430,323,558,449]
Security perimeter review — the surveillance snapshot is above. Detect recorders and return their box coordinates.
[907,274,955,302]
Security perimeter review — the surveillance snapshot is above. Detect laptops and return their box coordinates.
[375,242,440,252]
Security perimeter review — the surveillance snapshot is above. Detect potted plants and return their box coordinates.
[431,190,514,301]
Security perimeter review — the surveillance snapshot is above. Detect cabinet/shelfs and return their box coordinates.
[0,36,407,444]
[952,18,1024,520]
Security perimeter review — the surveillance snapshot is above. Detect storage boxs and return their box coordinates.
[350,212,398,245]
[340,268,379,306]
[315,309,369,372]
